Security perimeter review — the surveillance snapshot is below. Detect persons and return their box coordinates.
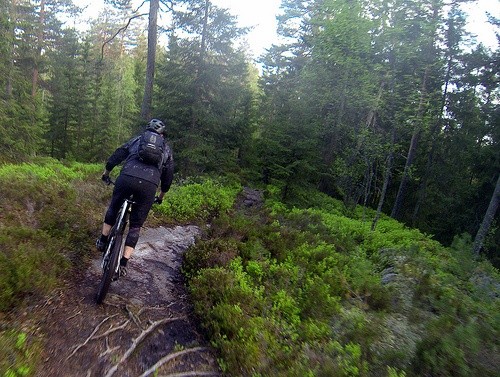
[95,119,176,278]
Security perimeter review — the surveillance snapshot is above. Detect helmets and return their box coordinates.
[145,118,166,134]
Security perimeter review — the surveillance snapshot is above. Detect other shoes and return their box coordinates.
[118,265,127,278]
[95,238,106,251]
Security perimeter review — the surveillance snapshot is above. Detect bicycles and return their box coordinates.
[96,173,162,306]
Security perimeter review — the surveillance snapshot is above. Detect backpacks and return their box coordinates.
[135,131,167,167]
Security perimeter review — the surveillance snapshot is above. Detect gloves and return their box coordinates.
[101,174,112,186]
[153,196,162,205]
